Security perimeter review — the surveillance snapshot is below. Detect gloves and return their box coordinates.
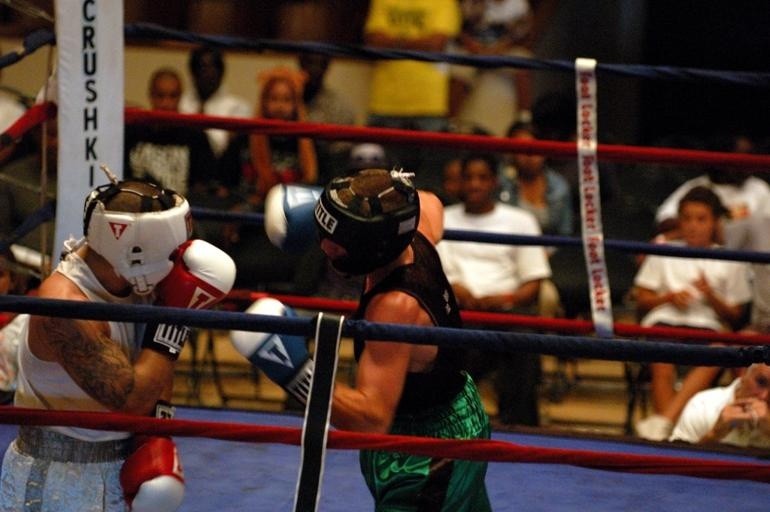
[264,181,326,252]
[142,238,238,363]
[119,399,185,512]
[230,296,315,403]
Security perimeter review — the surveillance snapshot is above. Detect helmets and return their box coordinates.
[82,175,194,297]
[311,162,422,277]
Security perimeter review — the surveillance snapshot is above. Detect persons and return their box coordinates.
[0,160,239,511]
[624,187,752,445]
[664,363,770,453]
[231,165,496,510]
